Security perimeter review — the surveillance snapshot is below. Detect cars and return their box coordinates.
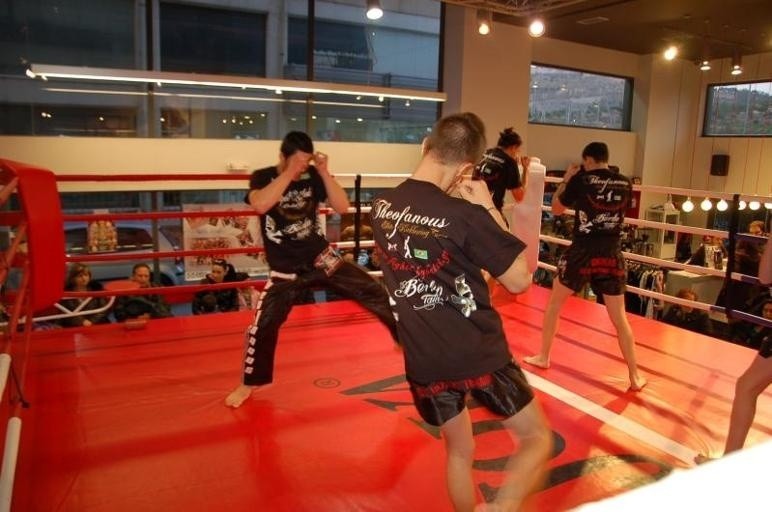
[1,222,186,293]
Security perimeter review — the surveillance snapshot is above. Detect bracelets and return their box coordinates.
[488,207,499,212]
[558,182,566,186]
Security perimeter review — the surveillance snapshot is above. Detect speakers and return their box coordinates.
[711,155,728,176]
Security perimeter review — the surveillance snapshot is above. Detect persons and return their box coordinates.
[341,214,373,240]
[694,222,772,467]
[472,127,531,284]
[112,263,174,323]
[371,111,554,512]
[664,289,707,337]
[61,265,111,326]
[226,132,402,408]
[191,260,314,314]
[326,249,380,303]
[523,141,647,389]
